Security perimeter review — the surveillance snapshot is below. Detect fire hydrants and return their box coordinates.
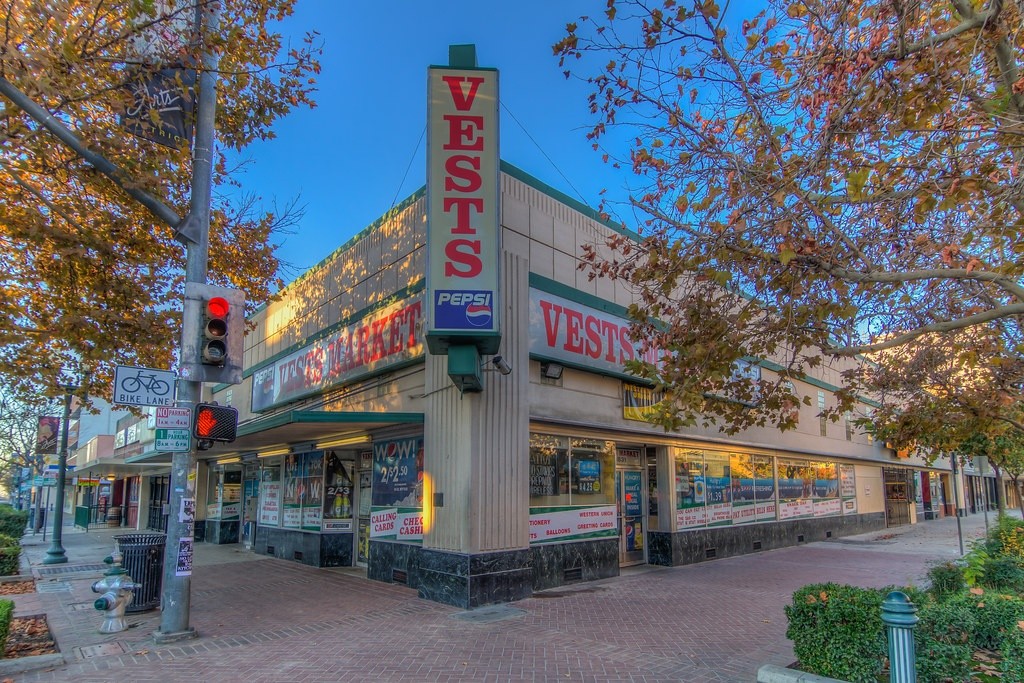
[90,552,142,633]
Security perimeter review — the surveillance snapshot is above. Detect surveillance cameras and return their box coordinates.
[492,355,513,376]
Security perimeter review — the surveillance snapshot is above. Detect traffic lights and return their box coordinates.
[192,403,238,442]
[200,297,230,367]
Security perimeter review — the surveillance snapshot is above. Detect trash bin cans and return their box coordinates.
[30,507,45,529]
[114,534,168,615]
